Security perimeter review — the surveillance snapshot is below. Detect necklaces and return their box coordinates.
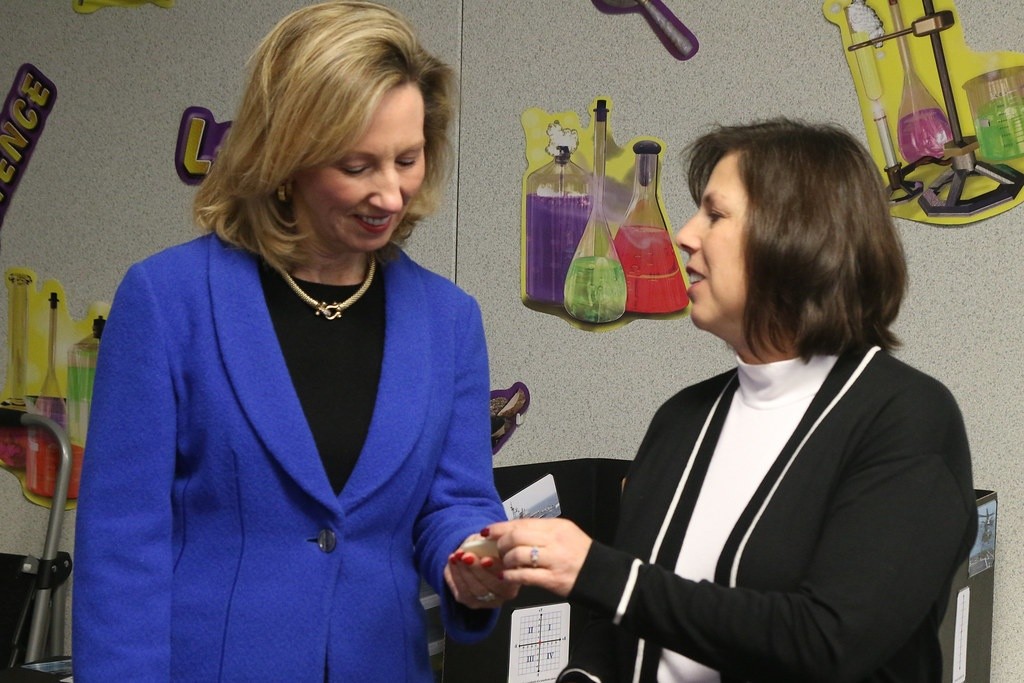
[281,253,376,320]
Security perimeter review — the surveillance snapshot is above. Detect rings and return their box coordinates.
[530,543,539,568]
[478,592,496,602]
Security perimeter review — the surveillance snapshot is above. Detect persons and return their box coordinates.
[480,113,979,683]
[72,2,519,681]
